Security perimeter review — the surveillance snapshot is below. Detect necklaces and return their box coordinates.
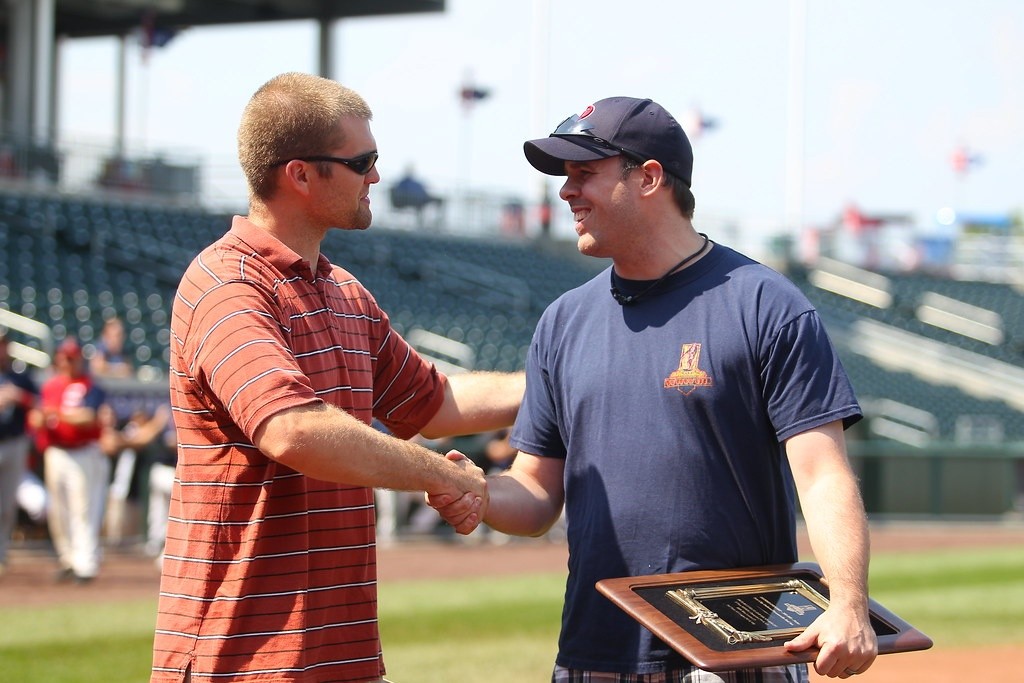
[609,231,709,305]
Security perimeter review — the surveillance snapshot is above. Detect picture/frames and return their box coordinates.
[595,562,935,675]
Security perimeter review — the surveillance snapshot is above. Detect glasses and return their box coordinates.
[549,114,648,164]
[270,151,378,175]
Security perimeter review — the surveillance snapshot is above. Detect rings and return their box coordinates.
[845,668,857,676]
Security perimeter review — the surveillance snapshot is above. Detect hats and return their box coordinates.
[524,96,693,188]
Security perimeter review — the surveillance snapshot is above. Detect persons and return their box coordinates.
[3,314,567,581]
[143,69,529,683]
[423,96,881,681]
